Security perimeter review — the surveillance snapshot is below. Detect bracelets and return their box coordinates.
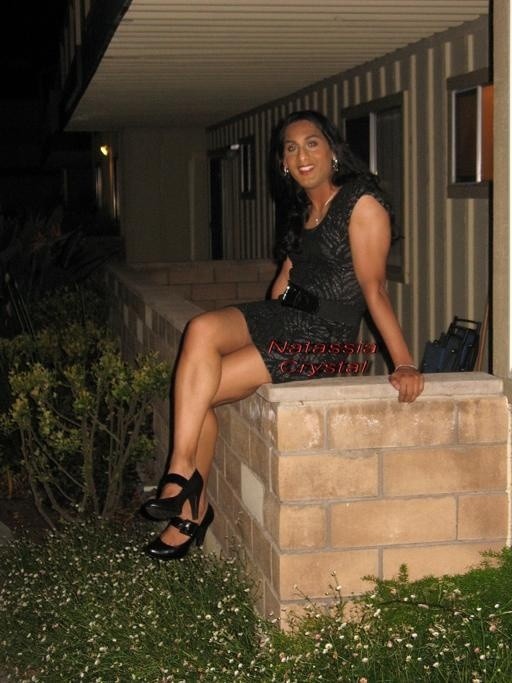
[392,363,419,372]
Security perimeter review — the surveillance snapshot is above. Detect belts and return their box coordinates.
[279,281,362,326]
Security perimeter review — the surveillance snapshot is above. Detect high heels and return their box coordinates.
[145,504,214,561]
[142,469,203,519]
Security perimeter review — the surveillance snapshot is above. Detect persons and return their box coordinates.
[142,107,425,562]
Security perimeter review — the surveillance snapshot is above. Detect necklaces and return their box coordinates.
[307,185,343,227]
